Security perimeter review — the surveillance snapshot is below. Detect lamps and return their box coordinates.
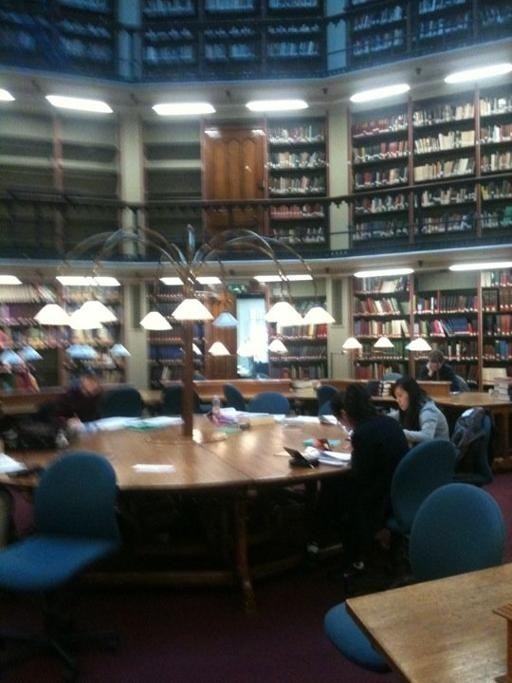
[306,299,334,326]
[370,332,394,369]
[38,302,71,326]
[139,308,173,333]
[75,299,119,325]
[343,340,360,362]
[407,333,432,367]
[62,308,99,329]
[173,298,214,324]
[266,300,302,328]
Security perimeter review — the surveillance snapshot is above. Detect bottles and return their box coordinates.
[213,395,220,416]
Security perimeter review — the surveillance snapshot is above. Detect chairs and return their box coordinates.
[247,386,291,416]
[0,449,124,680]
[450,412,497,489]
[105,384,142,414]
[322,482,510,670]
[160,384,198,419]
[220,382,246,410]
[314,383,341,417]
[371,437,465,579]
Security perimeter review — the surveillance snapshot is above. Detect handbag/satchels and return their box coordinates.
[450,428,478,473]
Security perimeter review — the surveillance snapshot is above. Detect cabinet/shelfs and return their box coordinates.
[342,0,512,73]
[134,0,328,80]
[349,107,412,244]
[481,269,512,386]
[481,87,512,241]
[261,117,331,251]
[411,91,477,243]
[269,293,328,379]
[349,273,410,383]
[60,284,124,394]
[0,282,63,395]
[0,0,122,76]
[148,285,209,382]
[139,114,202,260]
[416,273,480,386]
[0,130,123,260]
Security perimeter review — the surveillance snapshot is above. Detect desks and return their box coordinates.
[427,377,512,470]
[344,561,512,683]
[0,411,379,612]
[138,377,318,401]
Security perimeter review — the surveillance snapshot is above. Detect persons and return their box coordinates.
[309,381,409,551]
[60,372,103,431]
[420,350,461,391]
[388,376,449,444]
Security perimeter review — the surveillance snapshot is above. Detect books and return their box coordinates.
[352,141,408,165]
[270,123,322,142]
[354,317,407,334]
[151,326,181,338]
[479,95,512,115]
[151,303,180,316]
[481,206,512,229]
[268,341,325,358]
[269,151,325,168]
[271,227,324,243]
[414,104,475,126]
[358,361,405,381]
[269,175,325,192]
[355,220,409,240]
[482,181,512,199]
[417,318,475,334]
[450,365,479,380]
[150,346,184,361]
[352,113,408,138]
[358,277,410,291]
[355,297,408,314]
[355,167,408,190]
[481,152,512,173]
[358,340,409,356]
[482,289,512,312]
[293,298,326,317]
[414,130,474,153]
[414,187,476,208]
[417,340,476,357]
[416,214,472,234]
[483,315,511,335]
[270,204,324,217]
[490,269,512,287]
[285,324,327,338]
[481,124,512,144]
[482,339,512,360]
[355,194,410,215]
[151,366,186,381]
[415,156,475,182]
[275,362,325,379]
[417,294,473,310]
[482,365,512,382]
